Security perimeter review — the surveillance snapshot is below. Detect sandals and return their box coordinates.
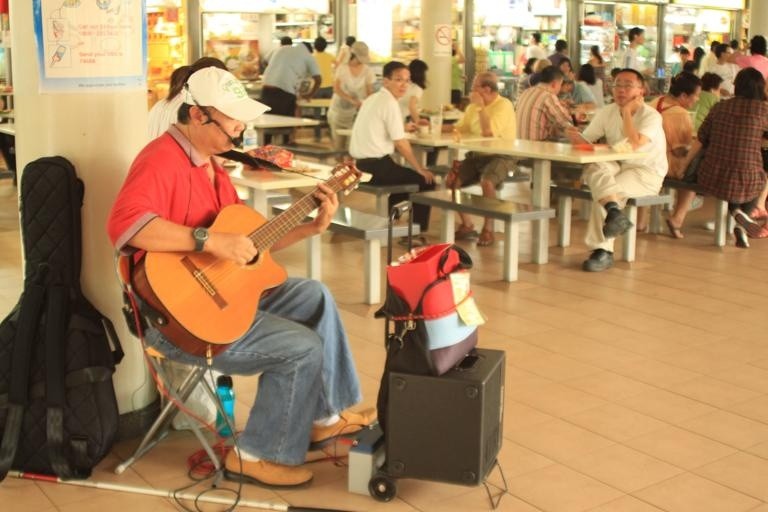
[454,224,476,240]
[476,228,494,247]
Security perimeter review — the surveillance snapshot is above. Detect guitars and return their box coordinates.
[131,163,364,356]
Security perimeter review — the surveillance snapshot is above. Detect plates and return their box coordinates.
[572,143,608,150]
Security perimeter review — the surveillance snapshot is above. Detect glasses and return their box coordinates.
[612,81,640,91]
[387,76,412,86]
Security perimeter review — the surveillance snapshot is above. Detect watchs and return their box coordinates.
[188,226,210,253]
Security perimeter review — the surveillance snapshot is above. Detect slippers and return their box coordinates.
[666,219,684,240]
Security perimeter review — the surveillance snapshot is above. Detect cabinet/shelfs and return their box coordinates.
[391,17,420,65]
[578,0,617,68]
[257,13,336,61]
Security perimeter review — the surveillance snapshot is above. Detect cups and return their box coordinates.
[418,116,443,139]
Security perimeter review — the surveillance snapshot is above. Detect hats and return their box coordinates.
[349,41,371,64]
[180,66,273,123]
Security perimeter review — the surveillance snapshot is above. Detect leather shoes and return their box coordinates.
[223,448,314,489]
[582,248,615,272]
[308,406,378,450]
[602,206,634,239]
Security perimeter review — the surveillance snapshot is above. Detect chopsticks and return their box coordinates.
[568,122,593,145]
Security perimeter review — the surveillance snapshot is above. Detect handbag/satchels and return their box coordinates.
[383,240,489,377]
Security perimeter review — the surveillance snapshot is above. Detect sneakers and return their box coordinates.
[704,205,768,239]
[733,224,751,248]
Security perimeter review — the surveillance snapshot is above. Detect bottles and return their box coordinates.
[657,65,665,78]
[243,124,258,153]
[215,376,235,436]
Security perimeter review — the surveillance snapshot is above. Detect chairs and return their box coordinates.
[113,252,226,490]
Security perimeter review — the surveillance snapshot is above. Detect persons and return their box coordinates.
[570,69,668,271]
[104,65,382,493]
[147,57,230,175]
[259,27,767,246]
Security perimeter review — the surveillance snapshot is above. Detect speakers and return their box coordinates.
[386,347,506,487]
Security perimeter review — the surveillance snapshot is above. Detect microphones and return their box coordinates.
[210,116,241,148]
